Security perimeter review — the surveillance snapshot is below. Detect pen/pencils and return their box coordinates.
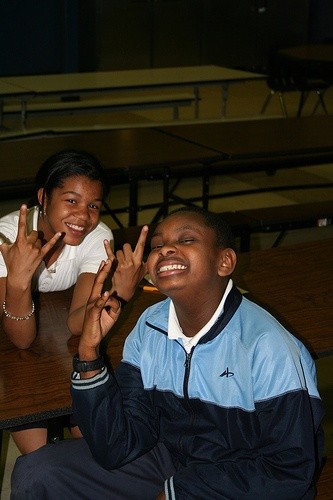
[138,284,159,292]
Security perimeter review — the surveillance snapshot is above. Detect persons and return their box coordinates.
[11,207,321,499]
[0,159,153,348]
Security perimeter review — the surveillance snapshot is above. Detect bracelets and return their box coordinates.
[72,352,107,374]
[2,299,36,321]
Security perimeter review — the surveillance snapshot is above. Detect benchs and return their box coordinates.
[113,201,250,253]
[235,201,333,247]
[2,92,201,120]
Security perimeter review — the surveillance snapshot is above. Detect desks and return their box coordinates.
[0,66,333,445]
